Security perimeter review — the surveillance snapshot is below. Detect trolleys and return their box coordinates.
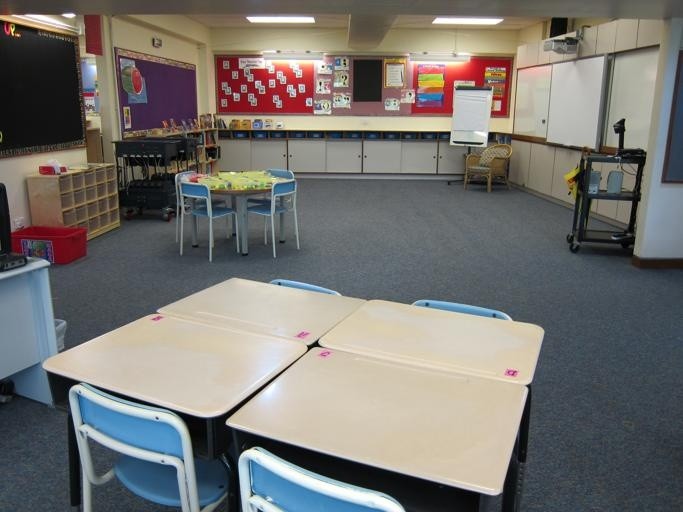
[153,134,201,212]
[111,137,180,222]
[566,145,646,255]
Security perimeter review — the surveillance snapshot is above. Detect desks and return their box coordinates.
[0,256,58,408]
[225,346,534,511]
[40,310,309,421]
[319,298,546,387]
[158,278,365,348]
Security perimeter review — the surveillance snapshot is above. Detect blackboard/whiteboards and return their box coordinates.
[1,19,87,164]
[353,59,382,102]
[511,43,660,157]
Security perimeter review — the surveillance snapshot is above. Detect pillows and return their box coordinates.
[479,148,508,167]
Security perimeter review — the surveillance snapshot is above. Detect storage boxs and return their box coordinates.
[11,225,88,265]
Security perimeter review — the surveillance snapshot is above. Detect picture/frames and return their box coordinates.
[661,50,683,184]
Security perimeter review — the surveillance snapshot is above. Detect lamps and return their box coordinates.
[410,29,470,62]
[260,27,324,62]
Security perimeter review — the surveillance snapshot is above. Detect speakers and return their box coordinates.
[607,171,623,193]
[588,171,601,194]
[550,18,567,37]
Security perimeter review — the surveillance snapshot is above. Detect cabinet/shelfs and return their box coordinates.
[160,128,220,174]
[250,139,326,173]
[27,163,121,241]
[326,138,401,175]
[402,141,467,174]
[216,140,250,172]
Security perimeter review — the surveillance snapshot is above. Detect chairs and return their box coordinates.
[237,445,407,512]
[63,381,228,509]
[269,276,345,296]
[411,297,515,319]
[174,168,300,263]
[464,144,512,193]
